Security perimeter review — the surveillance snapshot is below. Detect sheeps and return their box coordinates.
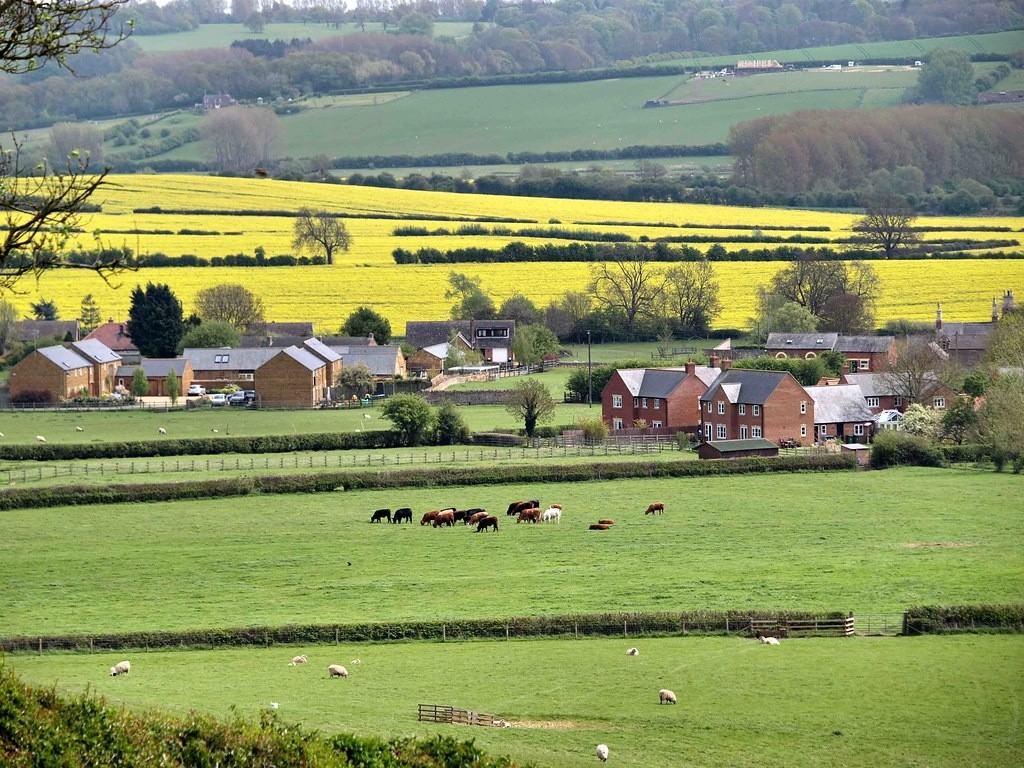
[292,655,308,666]
[110,661,130,676]
[759,636,780,645]
[597,744,609,762]
[363,413,371,418]
[212,429,217,432]
[626,648,639,655]
[37,435,47,442]
[328,664,348,678]
[659,689,676,705]
[158,428,167,434]
[76,427,84,433]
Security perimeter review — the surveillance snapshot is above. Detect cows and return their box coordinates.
[370,508,414,524]
[589,518,616,530]
[420,507,499,533]
[644,502,665,515]
[507,499,562,524]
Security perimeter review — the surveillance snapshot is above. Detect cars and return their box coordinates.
[189,384,207,396]
[228,389,255,406]
[212,394,228,406]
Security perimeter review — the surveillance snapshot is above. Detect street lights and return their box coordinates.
[587,330,593,407]
